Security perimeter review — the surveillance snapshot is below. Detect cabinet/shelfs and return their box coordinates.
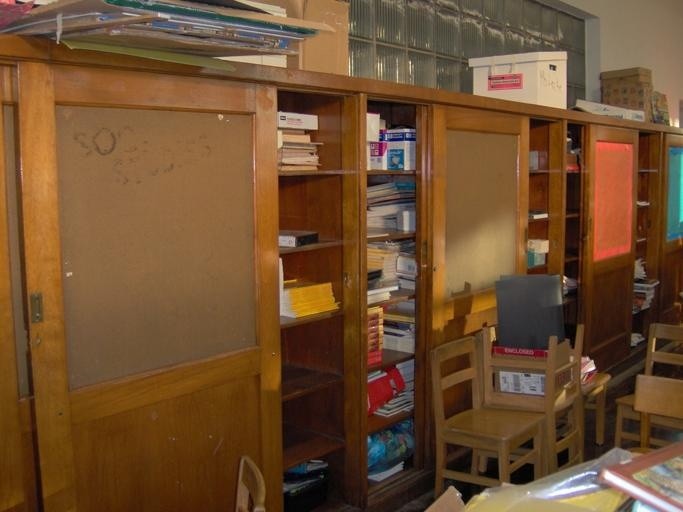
[0,37,683,512]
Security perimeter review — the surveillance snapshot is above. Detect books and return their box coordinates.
[570,355,599,387]
[278,129,325,172]
[367,181,417,482]
[278,228,320,248]
[463,439,682,512]
[633,278,660,315]
[283,459,329,495]
[282,278,341,319]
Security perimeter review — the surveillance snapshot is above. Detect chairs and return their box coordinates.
[634,374,683,451]
[615,322,683,446]
[481,325,587,476]
[428,335,544,501]
[583,374,614,445]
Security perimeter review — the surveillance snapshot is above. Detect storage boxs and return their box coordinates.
[467,51,569,111]
[600,67,653,120]
